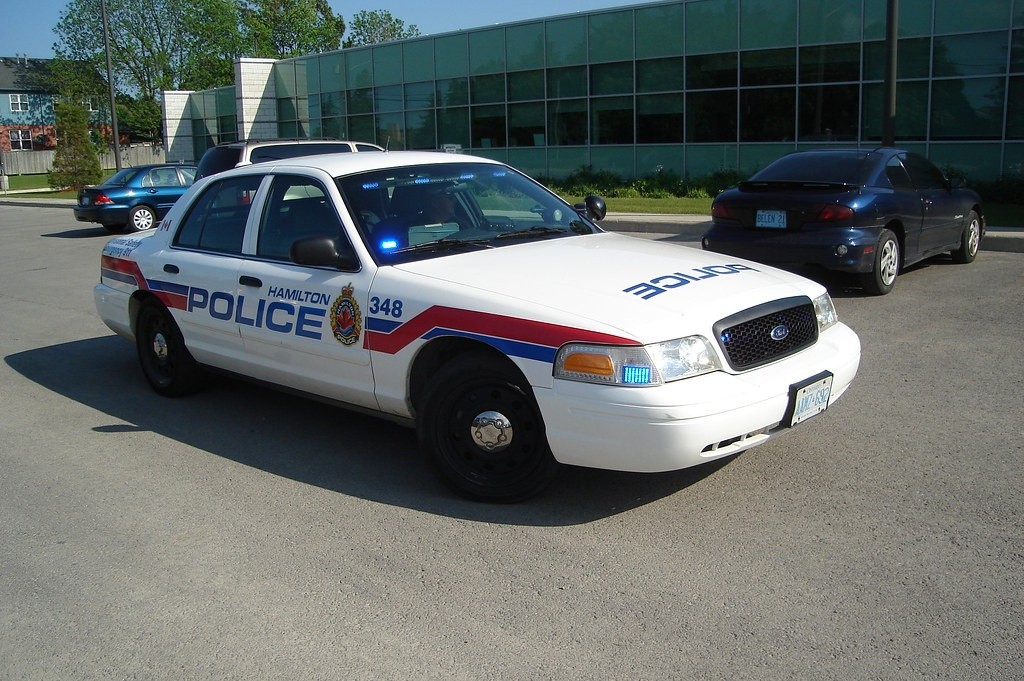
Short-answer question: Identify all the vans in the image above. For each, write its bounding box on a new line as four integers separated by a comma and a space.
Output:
190, 137, 401, 218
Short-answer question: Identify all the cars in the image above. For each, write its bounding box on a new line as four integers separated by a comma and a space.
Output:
701, 148, 986, 293
75, 163, 202, 233
92, 147, 864, 501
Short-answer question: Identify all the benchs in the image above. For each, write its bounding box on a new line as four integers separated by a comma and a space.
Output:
192, 199, 292, 252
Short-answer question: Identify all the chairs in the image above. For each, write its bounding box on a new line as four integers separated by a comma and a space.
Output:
152, 173, 164, 186
399, 194, 473, 246
165, 173, 179, 186
270, 198, 339, 267
